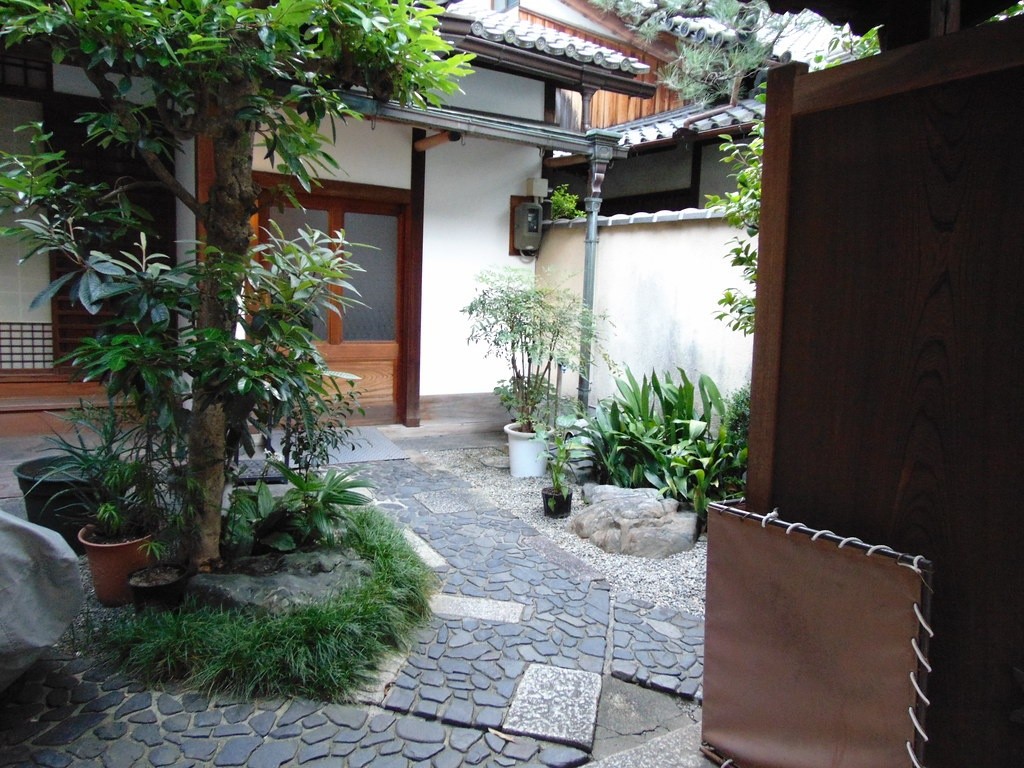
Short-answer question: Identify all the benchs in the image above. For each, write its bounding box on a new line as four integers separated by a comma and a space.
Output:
0, 394, 145, 436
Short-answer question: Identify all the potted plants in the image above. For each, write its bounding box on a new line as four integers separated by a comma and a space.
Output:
462, 264, 624, 478
14, 455, 111, 555
529, 424, 573, 519
40, 398, 153, 607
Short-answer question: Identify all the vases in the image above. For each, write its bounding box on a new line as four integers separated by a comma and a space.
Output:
127, 562, 188, 610
